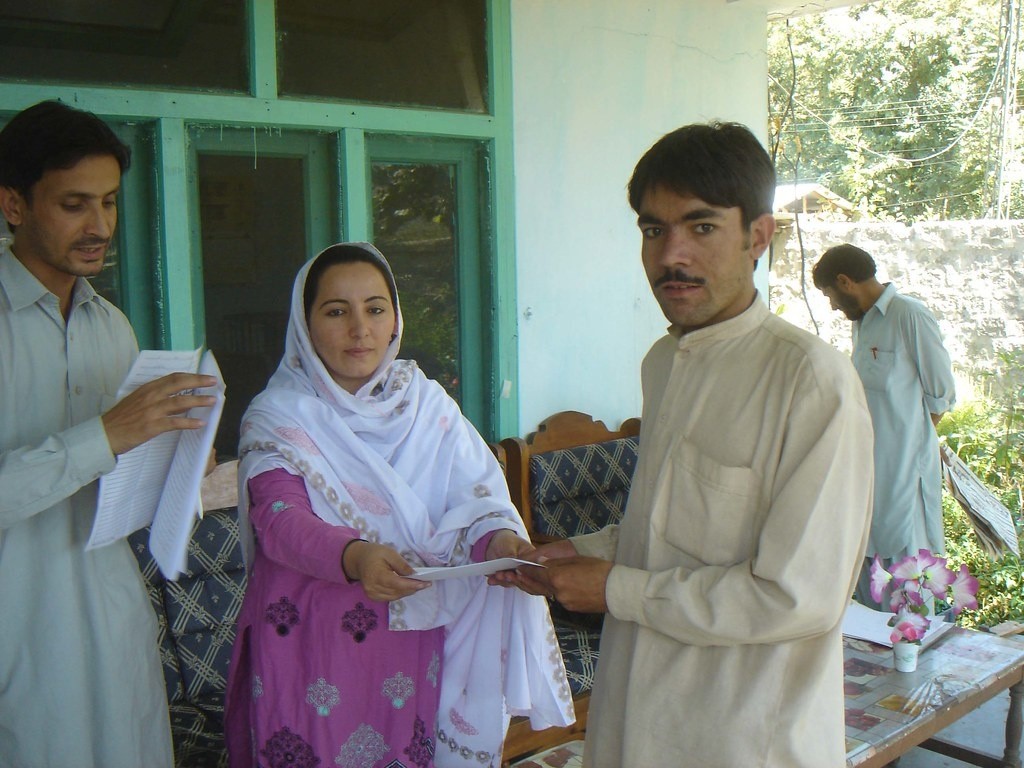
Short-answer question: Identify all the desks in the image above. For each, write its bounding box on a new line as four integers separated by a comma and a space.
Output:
507, 603, 1024, 768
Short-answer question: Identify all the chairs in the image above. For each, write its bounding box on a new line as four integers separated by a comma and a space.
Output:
129, 408, 641, 768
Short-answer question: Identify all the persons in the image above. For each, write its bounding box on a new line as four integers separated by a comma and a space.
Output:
222, 242, 535, 768
0, 98, 217, 768
508, 123, 875, 768
812, 242, 957, 625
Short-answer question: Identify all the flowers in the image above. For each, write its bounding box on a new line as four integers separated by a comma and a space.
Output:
869, 549, 982, 643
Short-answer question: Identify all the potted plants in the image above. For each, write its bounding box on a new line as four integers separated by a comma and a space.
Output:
890, 643, 922, 674
